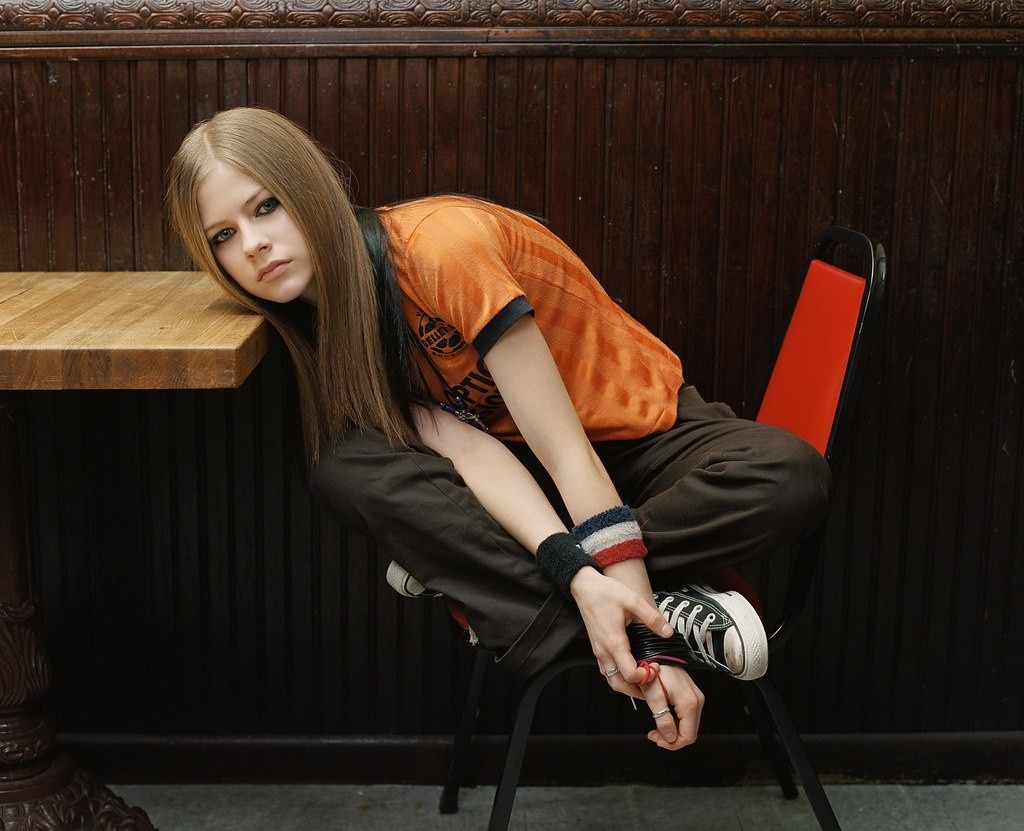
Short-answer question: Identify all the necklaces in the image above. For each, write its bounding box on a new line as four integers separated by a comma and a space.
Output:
407, 332, 489, 434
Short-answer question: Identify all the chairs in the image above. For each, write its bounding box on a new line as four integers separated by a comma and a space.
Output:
432, 222, 886, 831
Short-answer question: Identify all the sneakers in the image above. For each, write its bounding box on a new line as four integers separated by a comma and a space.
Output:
653, 583, 768, 680
387, 561, 444, 599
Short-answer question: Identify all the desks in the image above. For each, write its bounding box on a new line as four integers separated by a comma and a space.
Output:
0, 270, 270, 831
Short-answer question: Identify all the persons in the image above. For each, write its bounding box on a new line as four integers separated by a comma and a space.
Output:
168, 108, 831, 753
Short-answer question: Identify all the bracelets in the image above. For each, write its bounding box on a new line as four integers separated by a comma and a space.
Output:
535, 505, 649, 586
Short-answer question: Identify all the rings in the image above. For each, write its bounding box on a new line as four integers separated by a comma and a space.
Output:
604, 668, 619, 678
652, 708, 669, 719
